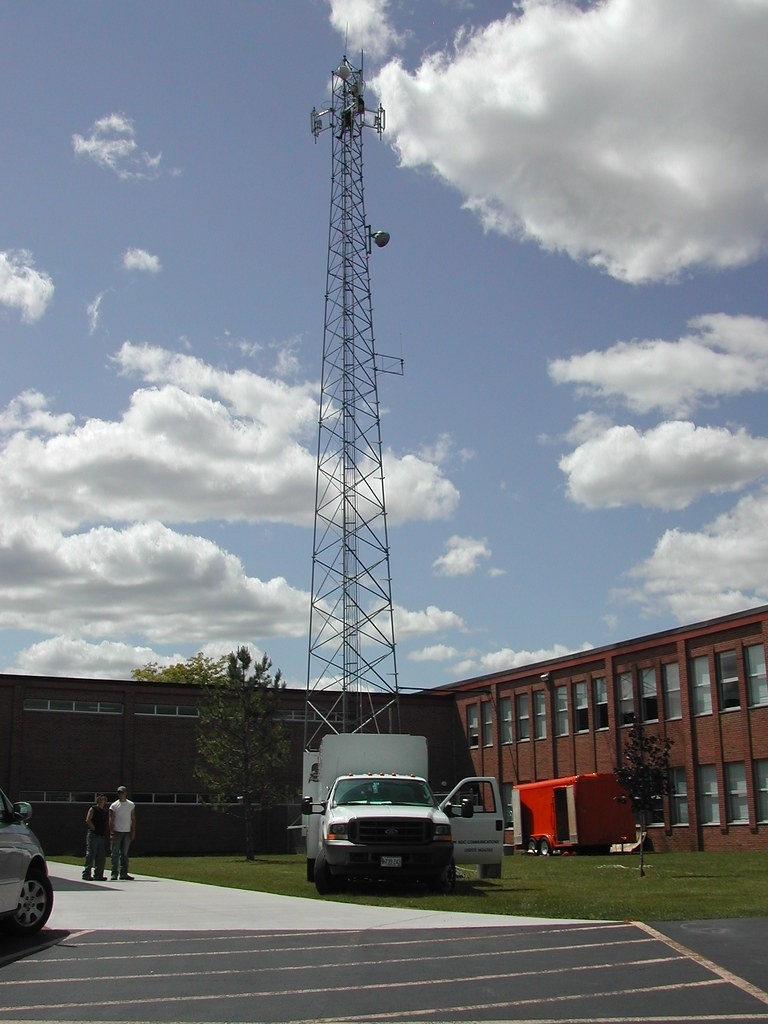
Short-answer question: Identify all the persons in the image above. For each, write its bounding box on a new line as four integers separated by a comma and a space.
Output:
82, 794, 109, 880
15, 805, 28, 819
108, 786, 135, 880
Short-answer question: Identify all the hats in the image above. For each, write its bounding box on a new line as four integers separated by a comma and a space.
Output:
97, 793, 105, 797
117, 786, 128, 792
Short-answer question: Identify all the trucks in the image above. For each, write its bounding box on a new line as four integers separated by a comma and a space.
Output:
301, 732, 505, 894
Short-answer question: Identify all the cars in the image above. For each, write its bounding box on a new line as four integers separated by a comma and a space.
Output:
0, 789, 53, 933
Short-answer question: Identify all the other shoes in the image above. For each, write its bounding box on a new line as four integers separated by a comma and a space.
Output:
82, 874, 94, 881
93, 875, 108, 881
111, 875, 117, 880
119, 873, 134, 881
